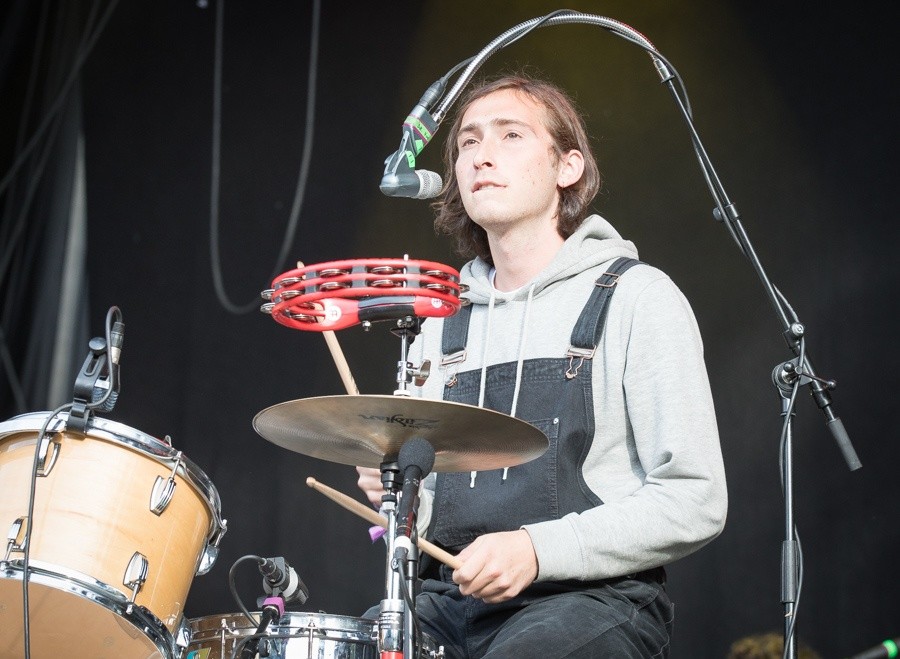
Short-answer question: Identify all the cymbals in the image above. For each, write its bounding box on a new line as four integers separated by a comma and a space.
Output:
259, 257, 472, 332
251, 394, 552, 475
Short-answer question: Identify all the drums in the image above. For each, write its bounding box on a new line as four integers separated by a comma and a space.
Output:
179, 609, 445, 659
0, 410, 229, 659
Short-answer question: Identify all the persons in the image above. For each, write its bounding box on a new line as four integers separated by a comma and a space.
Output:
357, 77, 728, 659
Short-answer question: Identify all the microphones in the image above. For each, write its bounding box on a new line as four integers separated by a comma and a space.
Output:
393, 436, 436, 563
857, 639, 900, 659
257, 557, 309, 607
380, 169, 443, 199
91, 320, 125, 412
